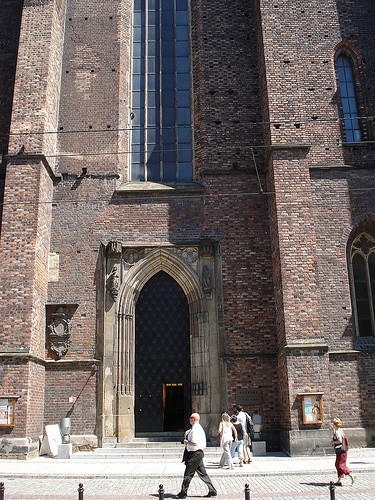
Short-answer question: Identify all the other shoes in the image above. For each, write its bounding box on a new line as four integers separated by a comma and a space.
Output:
334, 482, 342, 486
173, 495, 186, 499
204, 493, 217, 497
239, 460, 246, 464
239, 464, 243, 467
248, 459, 252, 463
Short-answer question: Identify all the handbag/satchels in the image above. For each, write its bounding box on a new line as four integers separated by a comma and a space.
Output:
217, 424, 223, 443
333, 436, 346, 454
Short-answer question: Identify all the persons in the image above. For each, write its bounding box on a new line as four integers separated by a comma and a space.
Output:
236, 405, 252, 464
203, 267, 211, 287
218, 413, 238, 469
172, 413, 217, 498
112, 267, 119, 289
331, 418, 355, 485
230, 415, 244, 467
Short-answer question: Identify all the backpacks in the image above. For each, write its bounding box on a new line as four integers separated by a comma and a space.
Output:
245, 412, 254, 436
